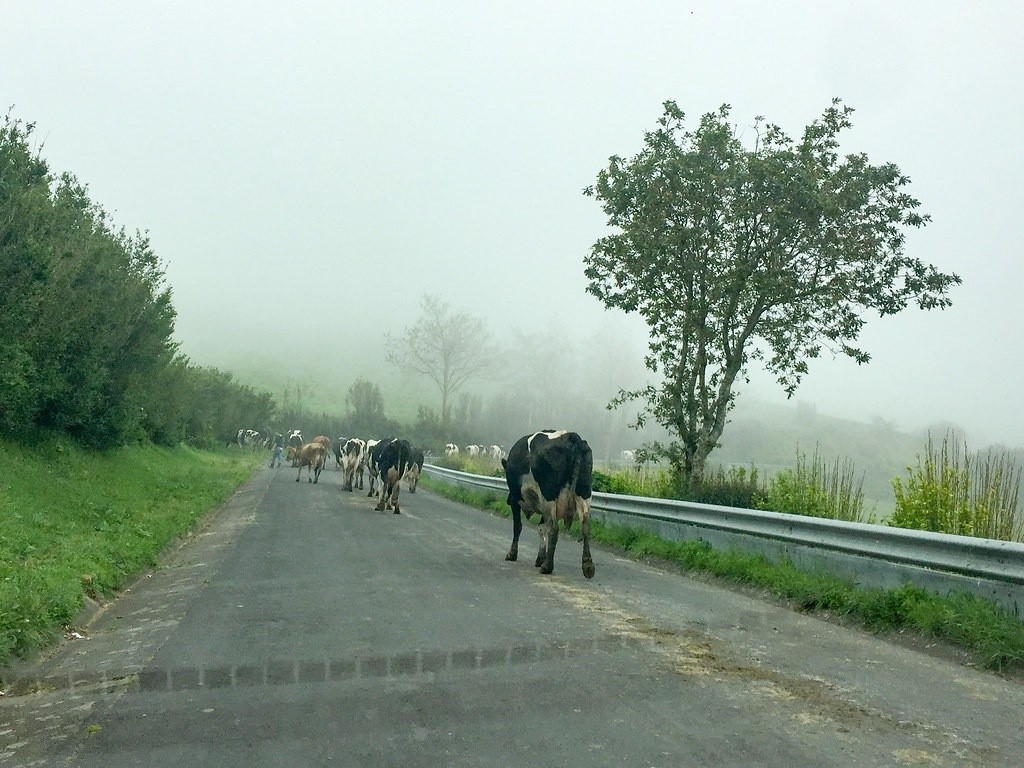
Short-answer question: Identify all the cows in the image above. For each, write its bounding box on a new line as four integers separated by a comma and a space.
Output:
621, 449, 635, 460
226, 428, 596, 579
